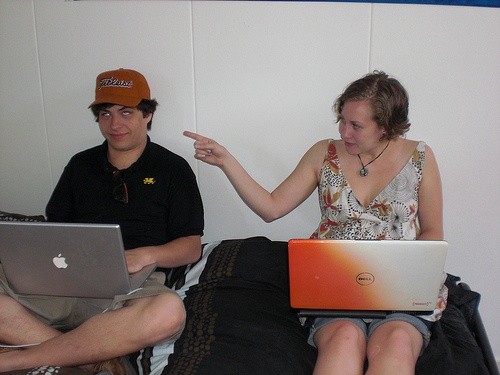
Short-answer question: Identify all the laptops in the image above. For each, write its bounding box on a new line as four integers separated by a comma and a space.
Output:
287, 239, 449, 319
0, 221, 159, 299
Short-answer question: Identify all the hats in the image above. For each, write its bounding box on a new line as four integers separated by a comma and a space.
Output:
88, 68, 150, 108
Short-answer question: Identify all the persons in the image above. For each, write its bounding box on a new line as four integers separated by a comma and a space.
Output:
0, 68, 204, 375
183, 71, 449, 375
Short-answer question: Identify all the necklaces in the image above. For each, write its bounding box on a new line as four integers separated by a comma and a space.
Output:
357, 139, 390, 177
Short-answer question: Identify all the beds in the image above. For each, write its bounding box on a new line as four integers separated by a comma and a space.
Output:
1, 211, 500, 375
